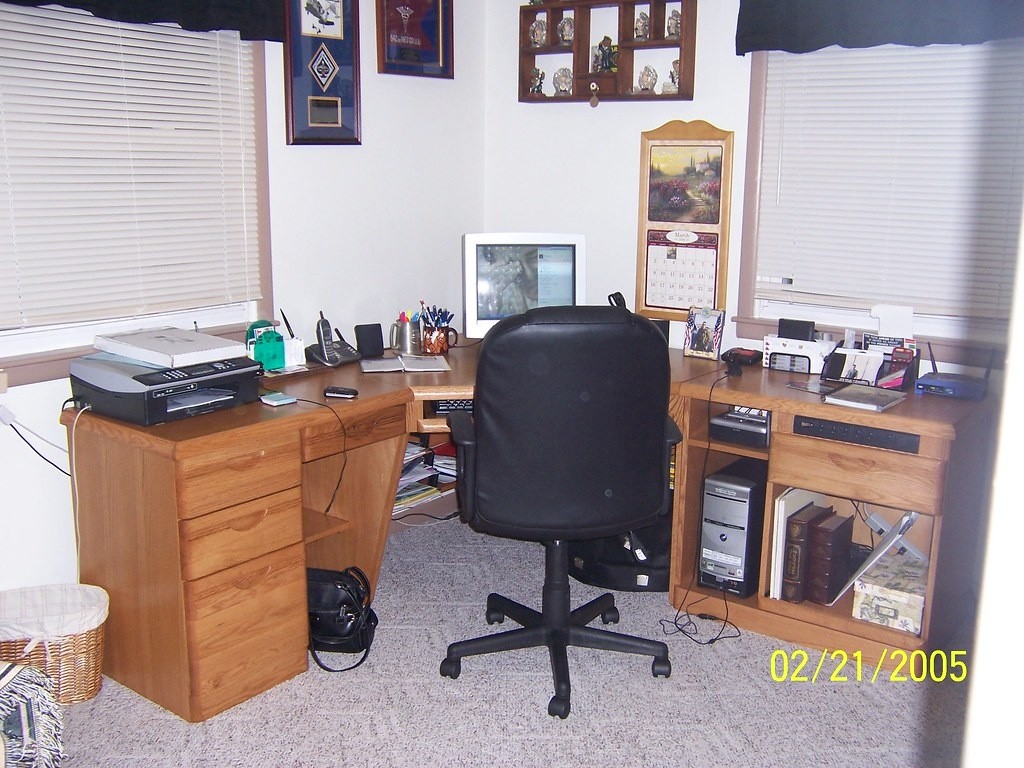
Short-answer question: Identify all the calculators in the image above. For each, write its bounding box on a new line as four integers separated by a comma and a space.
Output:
889, 347, 913, 374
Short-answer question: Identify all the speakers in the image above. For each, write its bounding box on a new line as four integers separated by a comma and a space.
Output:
355, 324, 384, 357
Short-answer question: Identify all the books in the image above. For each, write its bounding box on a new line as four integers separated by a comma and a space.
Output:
392, 442, 457, 516
359, 354, 452, 372
822, 383, 908, 413
769, 487, 855, 605
262, 392, 297, 406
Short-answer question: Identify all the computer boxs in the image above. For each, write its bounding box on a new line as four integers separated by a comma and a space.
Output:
696, 455, 769, 597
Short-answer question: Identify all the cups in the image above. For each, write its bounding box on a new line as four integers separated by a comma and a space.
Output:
423, 325, 458, 355
389, 319, 422, 355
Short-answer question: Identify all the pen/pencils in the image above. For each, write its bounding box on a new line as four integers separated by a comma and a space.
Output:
420, 300, 454, 327
280, 309, 295, 338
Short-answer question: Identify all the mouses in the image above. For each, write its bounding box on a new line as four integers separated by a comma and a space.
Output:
725, 359, 742, 376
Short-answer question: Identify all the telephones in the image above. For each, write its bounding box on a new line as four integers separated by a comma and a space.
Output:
304, 311, 362, 368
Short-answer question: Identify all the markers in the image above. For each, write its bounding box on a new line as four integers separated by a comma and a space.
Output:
399, 310, 420, 322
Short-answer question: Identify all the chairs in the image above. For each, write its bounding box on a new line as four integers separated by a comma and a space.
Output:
439, 305, 683, 720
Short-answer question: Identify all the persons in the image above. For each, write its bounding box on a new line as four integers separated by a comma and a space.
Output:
845, 364, 858, 379
694, 321, 709, 351
482, 245, 538, 318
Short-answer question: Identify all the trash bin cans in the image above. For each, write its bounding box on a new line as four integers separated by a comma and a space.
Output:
0, 585, 109, 706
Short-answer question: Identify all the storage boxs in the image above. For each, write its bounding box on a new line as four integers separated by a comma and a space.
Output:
762, 333, 835, 374
852, 552, 930, 636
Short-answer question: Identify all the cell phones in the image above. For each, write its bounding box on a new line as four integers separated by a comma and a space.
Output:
324, 386, 358, 398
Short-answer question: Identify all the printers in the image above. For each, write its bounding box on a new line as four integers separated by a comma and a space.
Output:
69, 350, 264, 427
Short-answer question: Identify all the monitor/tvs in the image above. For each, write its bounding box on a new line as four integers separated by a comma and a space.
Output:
462, 233, 587, 339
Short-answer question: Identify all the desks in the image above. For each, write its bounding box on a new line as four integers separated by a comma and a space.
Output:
332, 330, 728, 537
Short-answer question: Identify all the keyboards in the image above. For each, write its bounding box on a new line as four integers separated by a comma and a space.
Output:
432, 399, 474, 416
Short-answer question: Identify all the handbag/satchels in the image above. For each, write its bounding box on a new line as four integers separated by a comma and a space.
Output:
305, 565, 379, 673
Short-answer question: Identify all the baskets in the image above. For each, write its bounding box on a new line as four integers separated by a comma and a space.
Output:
0, 583, 110, 705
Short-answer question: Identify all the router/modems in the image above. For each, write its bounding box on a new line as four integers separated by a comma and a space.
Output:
914, 341, 996, 400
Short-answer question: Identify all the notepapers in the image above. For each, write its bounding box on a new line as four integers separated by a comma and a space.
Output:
259, 392, 297, 406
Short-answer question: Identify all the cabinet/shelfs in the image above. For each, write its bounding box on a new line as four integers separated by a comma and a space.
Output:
667, 359, 996, 679
59, 371, 416, 723
518, 0, 699, 108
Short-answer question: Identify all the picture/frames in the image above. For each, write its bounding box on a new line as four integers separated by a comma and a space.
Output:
375, 0, 455, 80
683, 306, 726, 360
283, 0, 363, 145
